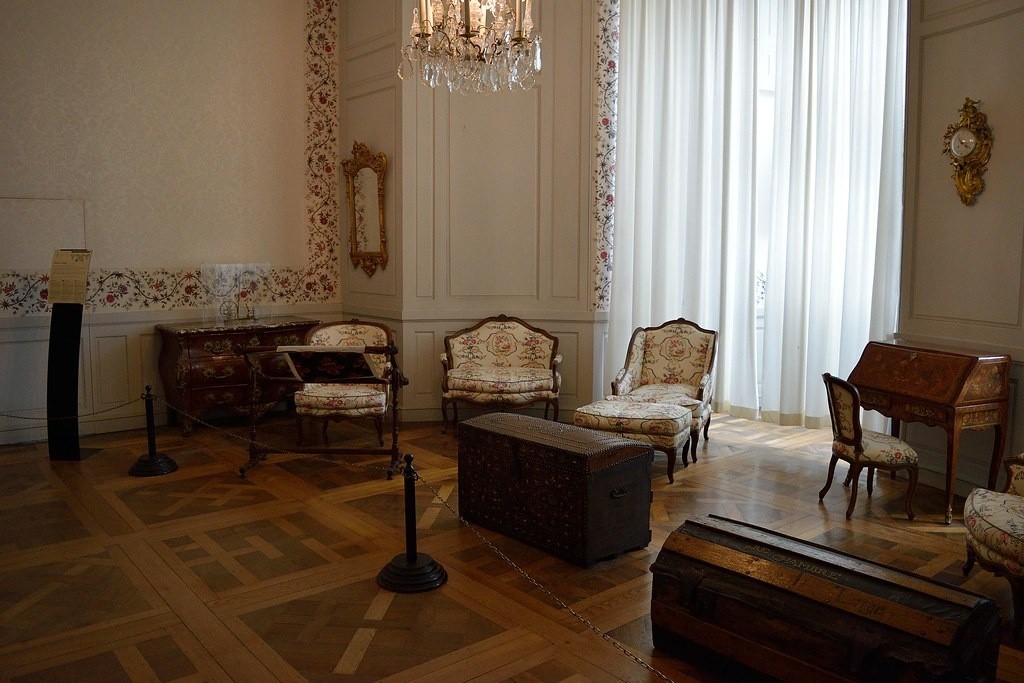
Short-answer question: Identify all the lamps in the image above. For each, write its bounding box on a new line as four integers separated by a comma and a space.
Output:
395, 0, 545, 94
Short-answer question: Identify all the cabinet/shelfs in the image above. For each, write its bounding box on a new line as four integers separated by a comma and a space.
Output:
155, 316, 320, 437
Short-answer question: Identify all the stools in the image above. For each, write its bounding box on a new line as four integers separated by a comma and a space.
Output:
574, 398, 694, 483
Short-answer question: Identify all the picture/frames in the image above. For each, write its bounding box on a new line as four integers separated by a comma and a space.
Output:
340, 139, 390, 277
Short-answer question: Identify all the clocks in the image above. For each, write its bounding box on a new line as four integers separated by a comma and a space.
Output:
940, 97, 993, 205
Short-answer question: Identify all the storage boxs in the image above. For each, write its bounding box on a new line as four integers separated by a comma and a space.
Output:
455, 409, 655, 570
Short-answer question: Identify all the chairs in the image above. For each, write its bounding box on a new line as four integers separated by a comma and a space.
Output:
963, 452, 1024, 641
294, 317, 394, 445
820, 372, 919, 522
610, 317, 719, 460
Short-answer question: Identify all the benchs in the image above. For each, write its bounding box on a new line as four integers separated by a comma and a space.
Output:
435, 314, 562, 433
